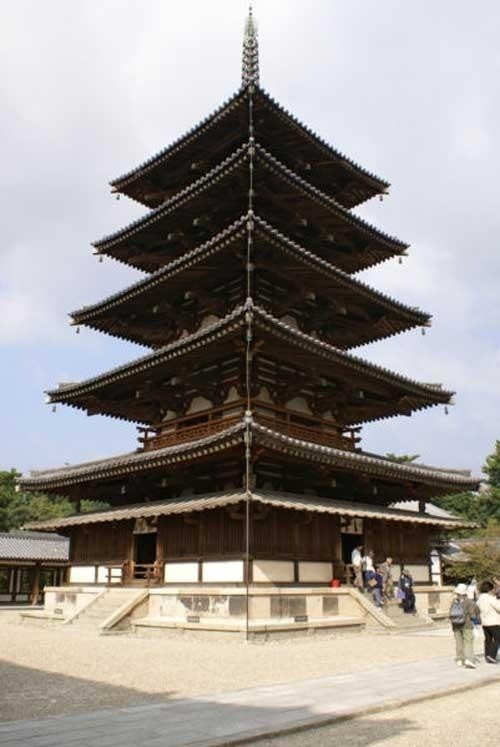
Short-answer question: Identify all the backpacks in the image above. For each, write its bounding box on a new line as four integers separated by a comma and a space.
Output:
449, 599, 466, 625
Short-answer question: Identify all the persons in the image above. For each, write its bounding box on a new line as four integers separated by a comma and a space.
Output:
448, 573, 500, 670
351, 541, 417, 614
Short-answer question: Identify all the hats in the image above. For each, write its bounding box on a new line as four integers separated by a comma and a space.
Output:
402, 568, 411, 575
454, 583, 468, 596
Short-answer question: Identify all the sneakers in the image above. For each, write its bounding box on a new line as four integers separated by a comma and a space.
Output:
457, 660, 475, 668
486, 655, 497, 663
